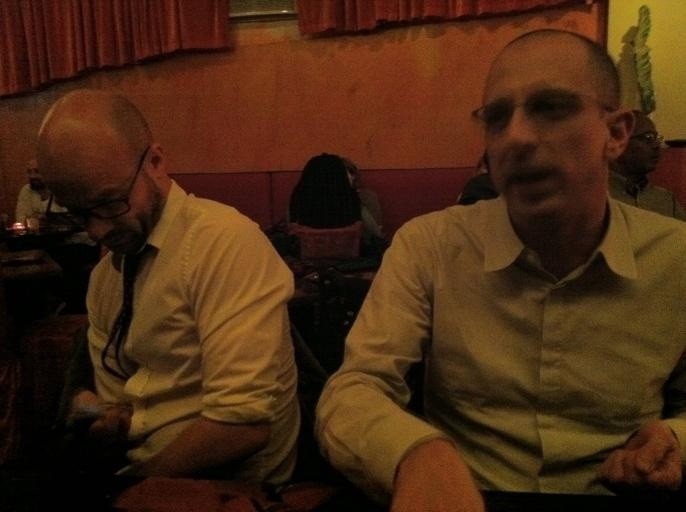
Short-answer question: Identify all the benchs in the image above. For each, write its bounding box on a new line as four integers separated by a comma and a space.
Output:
270, 166, 487, 248
646, 147, 686, 222
166, 172, 272, 235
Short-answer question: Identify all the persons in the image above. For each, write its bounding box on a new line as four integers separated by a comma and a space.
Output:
34, 89, 301, 511
313, 30, 686, 510
13, 157, 101, 264
604, 109, 684, 219
339, 152, 387, 254
451, 146, 499, 204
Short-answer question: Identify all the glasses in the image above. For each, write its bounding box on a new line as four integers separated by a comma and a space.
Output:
45, 148, 148, 227
470, 90, 610, 124
632, 132, 665, 145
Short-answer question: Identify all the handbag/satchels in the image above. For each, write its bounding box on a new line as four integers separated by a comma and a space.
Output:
288, 152, 358, 227
